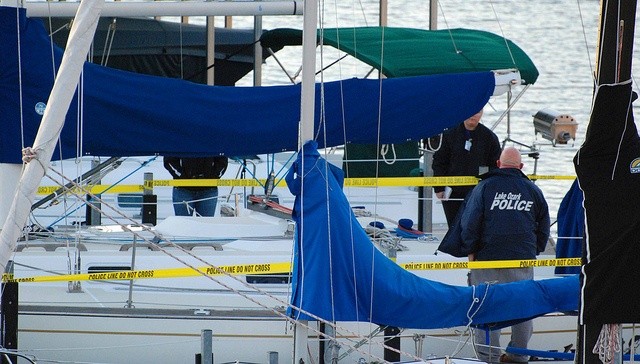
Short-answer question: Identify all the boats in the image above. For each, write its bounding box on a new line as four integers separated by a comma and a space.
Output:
0, 160, 640, 364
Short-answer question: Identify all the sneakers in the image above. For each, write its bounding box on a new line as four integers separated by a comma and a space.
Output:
500, 354, 528, 364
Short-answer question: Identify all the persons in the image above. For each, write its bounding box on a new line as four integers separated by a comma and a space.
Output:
162, 156, 228, 217
461, 147, 550, 363
433, 108, 502, 228
